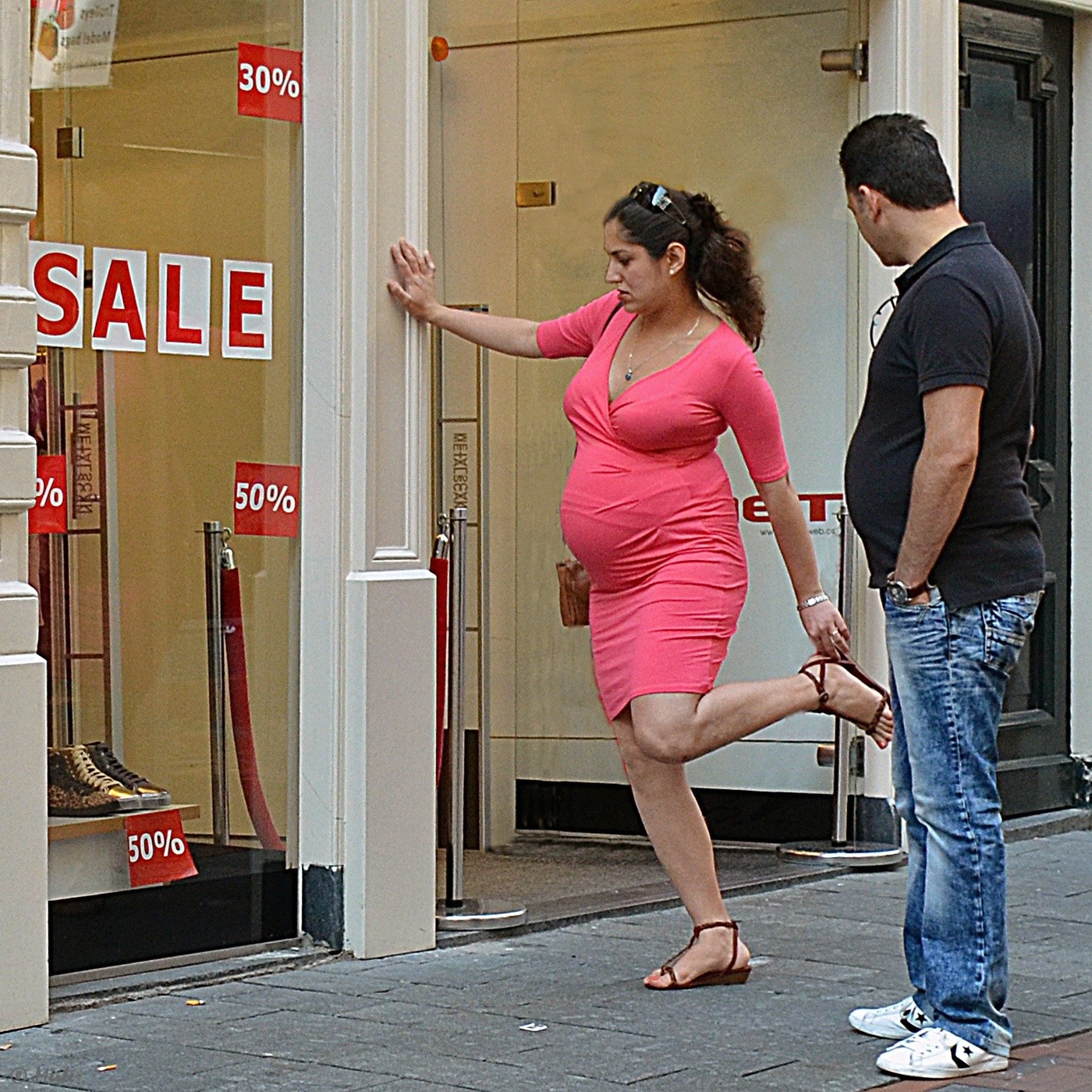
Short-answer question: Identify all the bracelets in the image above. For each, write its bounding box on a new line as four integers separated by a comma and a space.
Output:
796, 595, 829, 610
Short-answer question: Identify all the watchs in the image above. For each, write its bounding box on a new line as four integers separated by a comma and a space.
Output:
886, 571, 928, 604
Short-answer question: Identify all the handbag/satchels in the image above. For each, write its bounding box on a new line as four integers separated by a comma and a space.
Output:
556, 560, 590, 627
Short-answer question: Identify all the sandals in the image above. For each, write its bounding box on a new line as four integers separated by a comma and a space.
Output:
644, 920, 751, 990
799, 643, 894, 749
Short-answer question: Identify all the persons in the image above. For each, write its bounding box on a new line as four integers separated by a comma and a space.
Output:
385, 180, 892, 990
839, 112, 1046, 1080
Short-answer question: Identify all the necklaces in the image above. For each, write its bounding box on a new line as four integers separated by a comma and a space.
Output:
625, 314, 699, 380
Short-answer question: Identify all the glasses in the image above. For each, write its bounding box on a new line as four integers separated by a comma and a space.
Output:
630, 181, 693, 247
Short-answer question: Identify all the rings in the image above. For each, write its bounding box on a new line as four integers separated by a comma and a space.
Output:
830, 630, 838, 635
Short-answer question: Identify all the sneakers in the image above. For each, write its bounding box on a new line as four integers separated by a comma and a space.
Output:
60, 745, 142, 812
84, 742, 171, 809
848, 996, 935, 1039
876, 1027, 1008, 1079
48, 748, 119, 818
